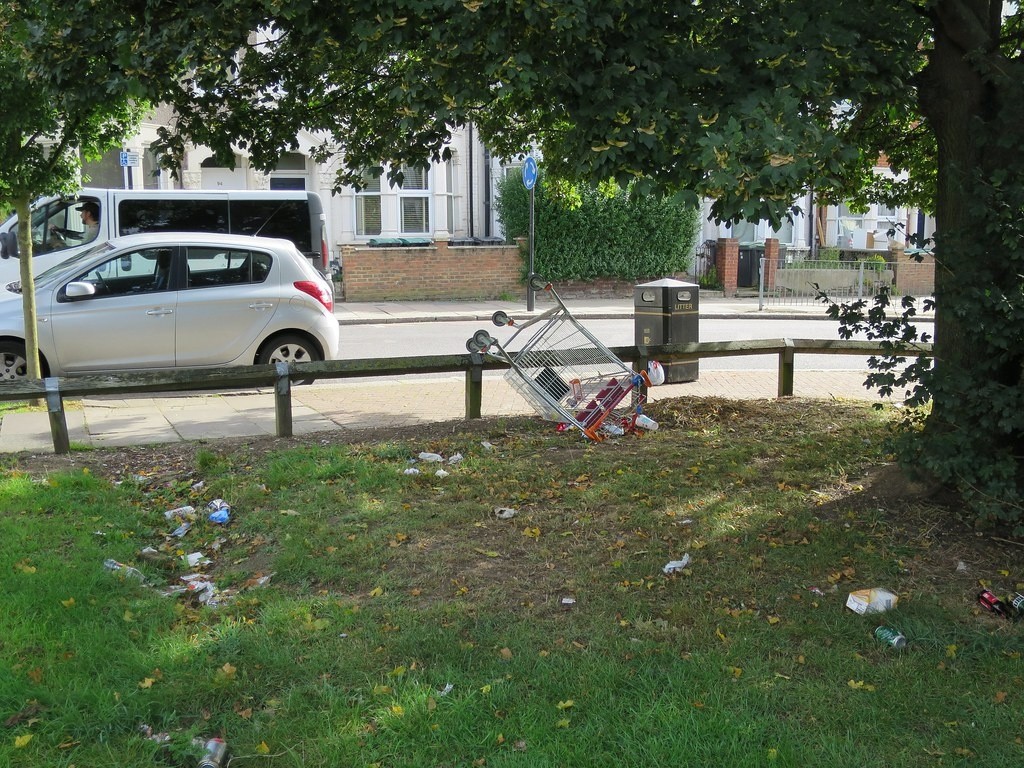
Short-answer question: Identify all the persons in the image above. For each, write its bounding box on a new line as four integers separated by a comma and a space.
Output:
128, 251, 172, 293
48, 202, 99, 249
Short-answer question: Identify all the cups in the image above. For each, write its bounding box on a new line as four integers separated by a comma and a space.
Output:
634, 414, 659, 430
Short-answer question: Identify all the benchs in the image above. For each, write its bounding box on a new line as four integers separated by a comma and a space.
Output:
233, 262, 267, 283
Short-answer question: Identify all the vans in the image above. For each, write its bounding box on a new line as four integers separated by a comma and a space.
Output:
0, 187, 335, 297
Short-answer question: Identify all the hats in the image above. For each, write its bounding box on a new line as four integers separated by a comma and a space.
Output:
75, 203, 99, 214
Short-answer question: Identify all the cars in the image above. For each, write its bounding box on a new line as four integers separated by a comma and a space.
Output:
0, 231, 338, 388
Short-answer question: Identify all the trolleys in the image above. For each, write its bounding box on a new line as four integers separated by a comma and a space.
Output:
465, 270, 652, 447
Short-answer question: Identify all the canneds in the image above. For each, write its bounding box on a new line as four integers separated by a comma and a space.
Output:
196, 737, 228, 768
1005, 590, 1024, 615
875, 625, 906, 648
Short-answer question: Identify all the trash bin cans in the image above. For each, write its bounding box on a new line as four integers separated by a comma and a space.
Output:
633, 277, 699, 383
366, 235, 505, 247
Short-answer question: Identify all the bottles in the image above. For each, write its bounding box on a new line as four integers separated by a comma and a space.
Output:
1006, 592, 1024, 616
103, 558, 146, 585
976, 589, 1015, 621
